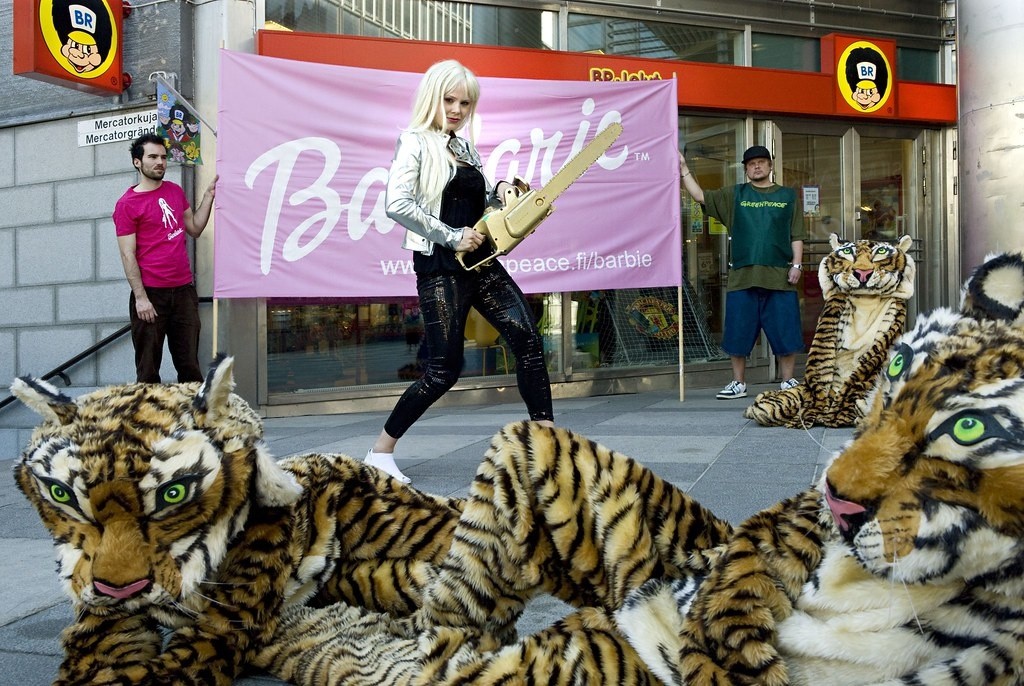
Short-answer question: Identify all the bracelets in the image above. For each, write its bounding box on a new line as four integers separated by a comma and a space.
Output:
793, 264, 804, 271
681, 171, 691, 177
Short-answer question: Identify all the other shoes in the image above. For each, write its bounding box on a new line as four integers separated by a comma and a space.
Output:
361, 448, 411, 484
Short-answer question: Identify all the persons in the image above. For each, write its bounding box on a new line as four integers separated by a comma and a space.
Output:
678, 146, 811, 400
111, 135, 220, 385
363, 58, 554, 484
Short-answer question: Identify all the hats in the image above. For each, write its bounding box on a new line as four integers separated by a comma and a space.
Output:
742, 146, 771, 162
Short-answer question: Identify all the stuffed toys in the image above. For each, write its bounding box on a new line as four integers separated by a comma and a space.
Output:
10, 352, 532, 685
744, 228, 920, 431
393, 253, 1024, 685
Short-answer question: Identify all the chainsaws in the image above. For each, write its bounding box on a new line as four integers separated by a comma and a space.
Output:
453, 122, 625, 273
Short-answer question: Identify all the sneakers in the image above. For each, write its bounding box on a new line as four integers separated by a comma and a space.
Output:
716, 380, 748, 399
779, 377, 799, 390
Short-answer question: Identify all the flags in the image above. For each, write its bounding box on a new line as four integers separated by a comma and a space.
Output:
154, 81, 204, 166
